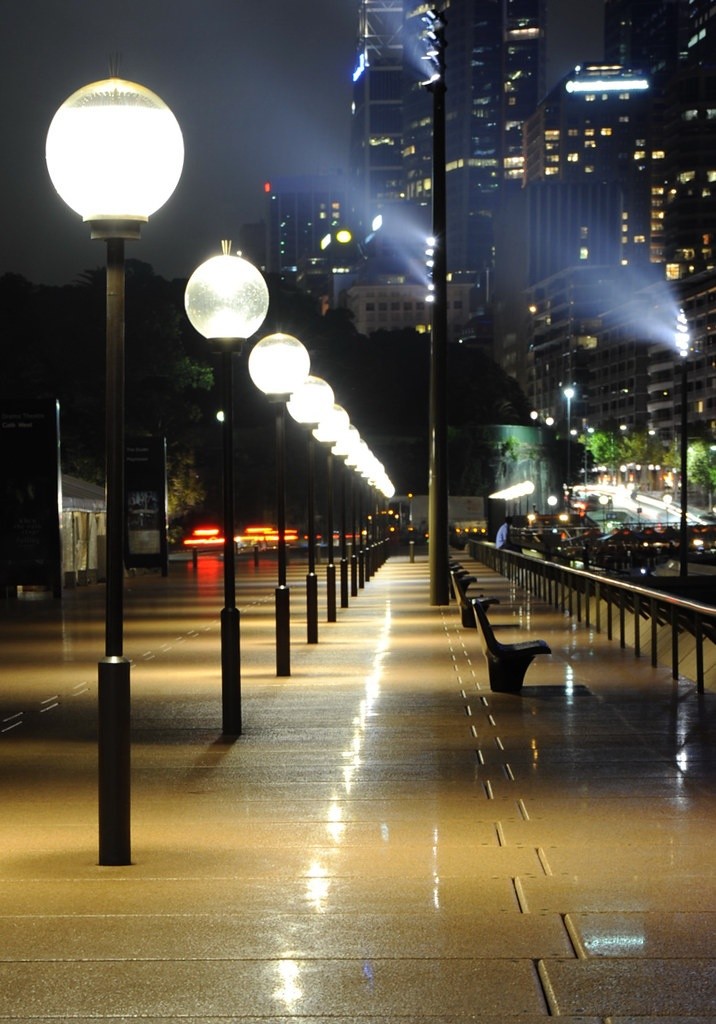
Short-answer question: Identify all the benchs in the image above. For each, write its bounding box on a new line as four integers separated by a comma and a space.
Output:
448, 551, 552, 693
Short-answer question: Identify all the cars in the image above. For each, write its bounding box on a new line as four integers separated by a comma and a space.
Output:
673, 524, 716, 562
510, 513, 600, 560
585, 528, 677, 573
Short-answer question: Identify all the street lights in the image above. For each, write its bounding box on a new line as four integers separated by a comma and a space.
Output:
563, 388, 574, 521
599, 496, 609, 534
679, 349, 693, 583
248, 332, 311, 678
547, 496, 557, 521
183, 239, 271, 733
663, 494, 672, 528
286, 374, 395, 643
488, 482, 535, 516
45, 78, 184, 869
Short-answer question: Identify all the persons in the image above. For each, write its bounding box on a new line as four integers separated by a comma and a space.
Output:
495, 516, 521, 553
